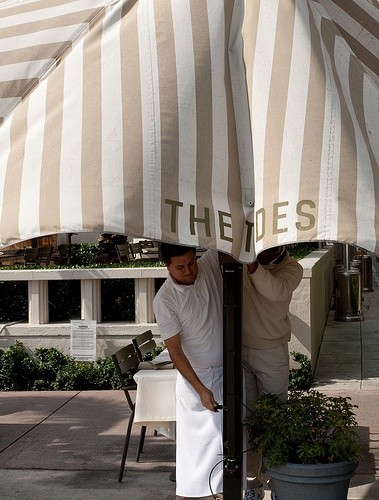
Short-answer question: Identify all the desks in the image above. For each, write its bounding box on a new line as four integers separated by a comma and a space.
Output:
141, 246, 207, 263
133, 348, 177, 483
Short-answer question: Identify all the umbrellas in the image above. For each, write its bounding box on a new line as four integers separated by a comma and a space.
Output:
0, 0, 379, 500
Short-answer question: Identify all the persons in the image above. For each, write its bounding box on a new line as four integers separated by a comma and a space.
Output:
151, 243, 226, 500
244, 245, 303, 500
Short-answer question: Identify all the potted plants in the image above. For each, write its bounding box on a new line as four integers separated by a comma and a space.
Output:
242, 389, 364, 500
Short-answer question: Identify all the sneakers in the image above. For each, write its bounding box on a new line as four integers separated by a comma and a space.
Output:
270, 490, 280, 500
244, 484, 266, 500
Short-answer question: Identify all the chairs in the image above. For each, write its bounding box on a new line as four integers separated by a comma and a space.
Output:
111, 343, 147, 483
0, 239, 154, 267
132, 329, 158, 363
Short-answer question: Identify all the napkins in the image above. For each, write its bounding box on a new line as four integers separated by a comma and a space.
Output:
137, 361, 176, 370
